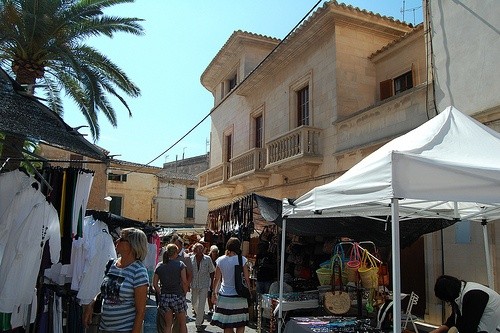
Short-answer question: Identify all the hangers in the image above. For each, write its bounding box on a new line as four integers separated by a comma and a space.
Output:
18, 161, 108, 222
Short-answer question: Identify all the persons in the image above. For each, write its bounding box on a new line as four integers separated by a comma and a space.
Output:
211, 237, 252, 333
83, 227, 150, 333
187, 242, 216, 332
146, 231, 210, 302
207, 245, 219, 315
254, 241, 278, 319
153, 244, 190, 333
262, 273, 293, 326
429, 275, 500, 333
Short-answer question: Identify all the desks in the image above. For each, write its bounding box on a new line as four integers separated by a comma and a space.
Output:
282, 317, 357, 333
261, 294, 410, 333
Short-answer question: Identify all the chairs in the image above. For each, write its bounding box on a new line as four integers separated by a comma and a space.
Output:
401, 291, 419, 333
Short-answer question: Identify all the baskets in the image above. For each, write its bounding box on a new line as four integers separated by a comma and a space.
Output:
316, 243, 390, 288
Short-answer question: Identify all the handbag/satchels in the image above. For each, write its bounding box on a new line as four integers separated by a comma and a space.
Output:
93, 293, 107, 314
323, 291, 351, 315
235, 255, 251, 298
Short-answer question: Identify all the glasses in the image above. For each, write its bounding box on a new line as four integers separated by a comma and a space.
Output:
119, 236, 128, 241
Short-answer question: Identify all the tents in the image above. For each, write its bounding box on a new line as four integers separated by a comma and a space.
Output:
277, 105, 500, 333
